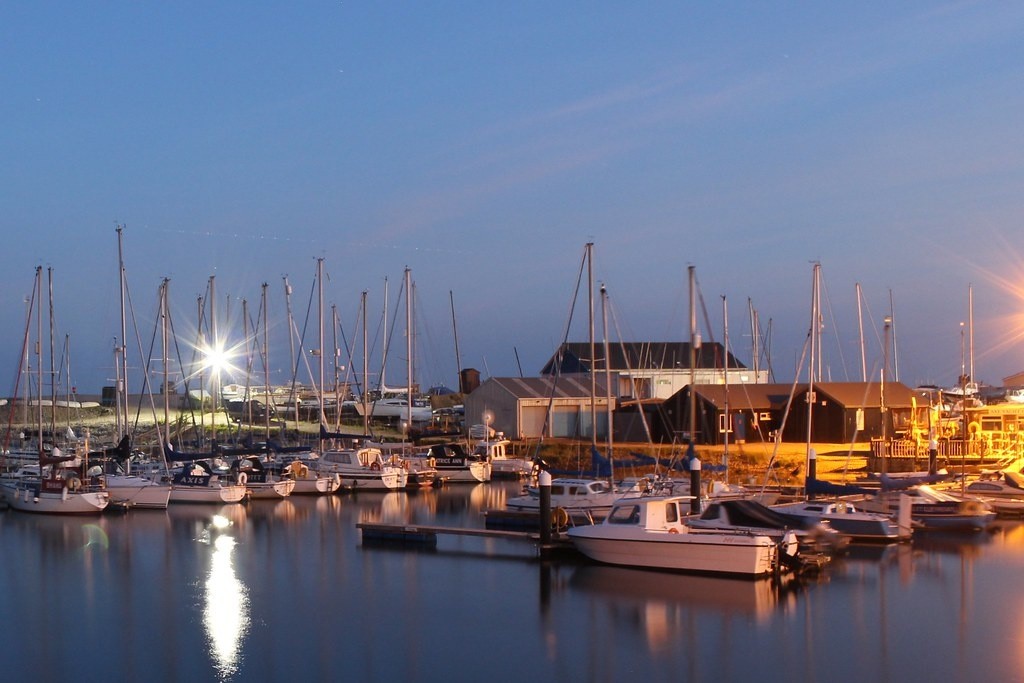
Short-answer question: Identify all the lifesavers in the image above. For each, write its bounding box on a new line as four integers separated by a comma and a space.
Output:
707, 478, 715, 494
238, 472, 247, 484
68, 477, 82, 490
670, 527, 681, 535
298, 467, 307, 478
371, 462, 380, 471
551, 507, 568, 526
429, 457, 436, 467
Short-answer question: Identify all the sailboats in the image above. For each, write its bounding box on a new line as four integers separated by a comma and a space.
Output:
0, 239, 1024, 574
